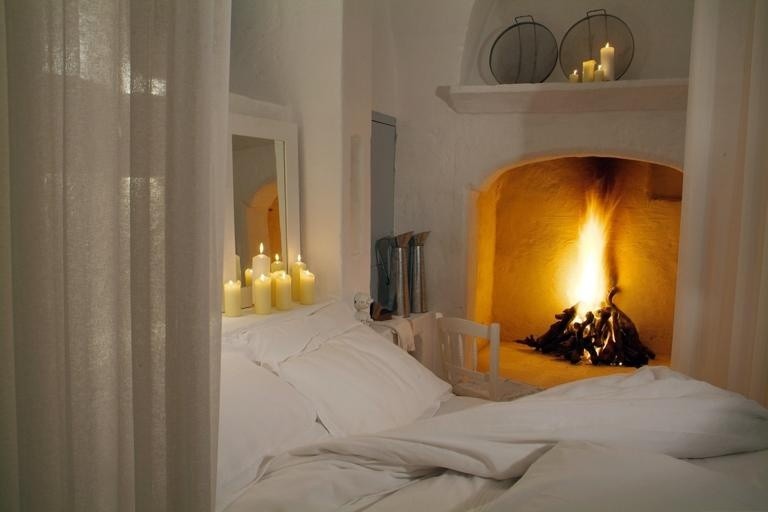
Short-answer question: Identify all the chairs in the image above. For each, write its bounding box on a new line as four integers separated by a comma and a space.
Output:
437, 312, 540, 401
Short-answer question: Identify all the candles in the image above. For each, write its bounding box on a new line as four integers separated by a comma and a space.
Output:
570, 42, 615, 82
224, 242, 316, 317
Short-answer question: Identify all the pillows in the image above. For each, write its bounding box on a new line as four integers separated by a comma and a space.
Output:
214, 350, 331, 509
224, 302, 363, 372
268, 323, 455, 440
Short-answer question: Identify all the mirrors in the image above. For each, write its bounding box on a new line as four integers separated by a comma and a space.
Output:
370, 120, 396, 311
224, 114, 300, 309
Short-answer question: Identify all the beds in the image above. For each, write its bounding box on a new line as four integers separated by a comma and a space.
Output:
214, 301, 768, 512
